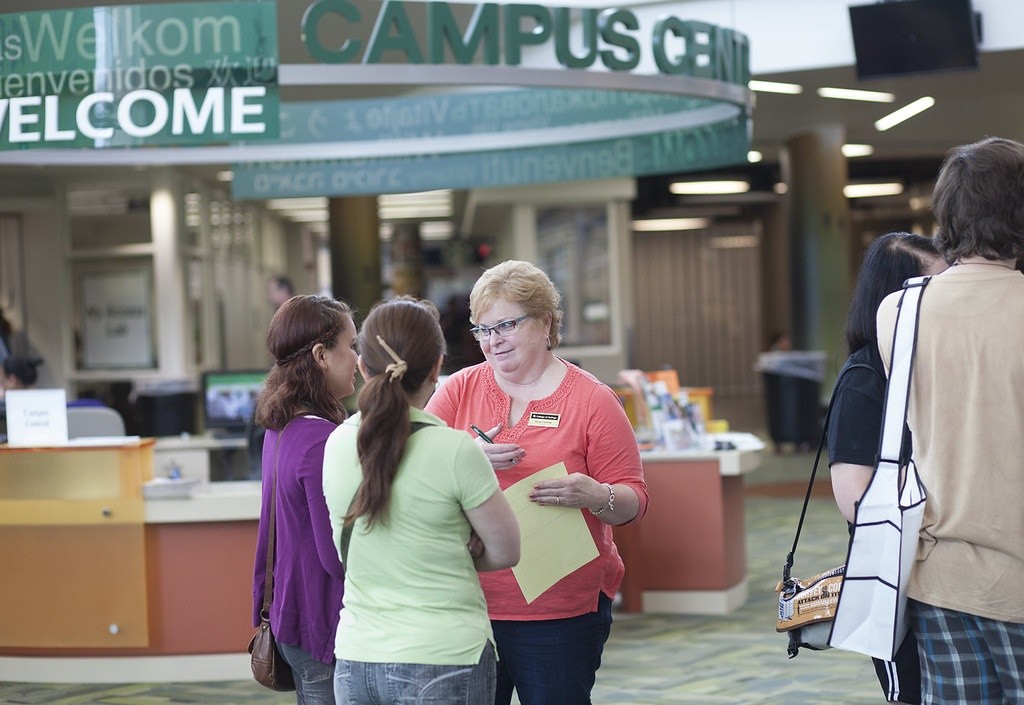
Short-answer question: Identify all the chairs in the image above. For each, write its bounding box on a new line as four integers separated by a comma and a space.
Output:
65, 407, 127, 437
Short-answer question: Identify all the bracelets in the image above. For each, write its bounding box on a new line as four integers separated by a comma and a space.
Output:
588, 483, 614, 515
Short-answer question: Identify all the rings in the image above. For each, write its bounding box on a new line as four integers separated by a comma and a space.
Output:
556, 497, 559, 504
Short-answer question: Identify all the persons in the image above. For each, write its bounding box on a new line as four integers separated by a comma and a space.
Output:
829, 137, 1024, 705
322, 295, 520, 705
253, 295, 358, 705
423, 260, 649, 705
0, 304, 39, 434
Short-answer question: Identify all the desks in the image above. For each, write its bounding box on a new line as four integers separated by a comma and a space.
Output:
151, 430, 250, 483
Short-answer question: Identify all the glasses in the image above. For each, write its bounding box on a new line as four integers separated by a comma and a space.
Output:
468, 312, 531, 341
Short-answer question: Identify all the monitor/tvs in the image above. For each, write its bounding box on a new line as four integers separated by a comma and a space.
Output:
201, 369, 269, 440
848, 0, 980, 78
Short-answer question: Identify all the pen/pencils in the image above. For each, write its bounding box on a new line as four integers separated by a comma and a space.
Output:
468, 424, 518, 466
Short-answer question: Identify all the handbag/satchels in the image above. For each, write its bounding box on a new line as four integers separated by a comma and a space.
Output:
246, 624, 297, 693
826, 463, 927, 663
775, 564, 845, 658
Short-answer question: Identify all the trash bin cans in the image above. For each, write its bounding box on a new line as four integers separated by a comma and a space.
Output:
754, 352, 825, 455
